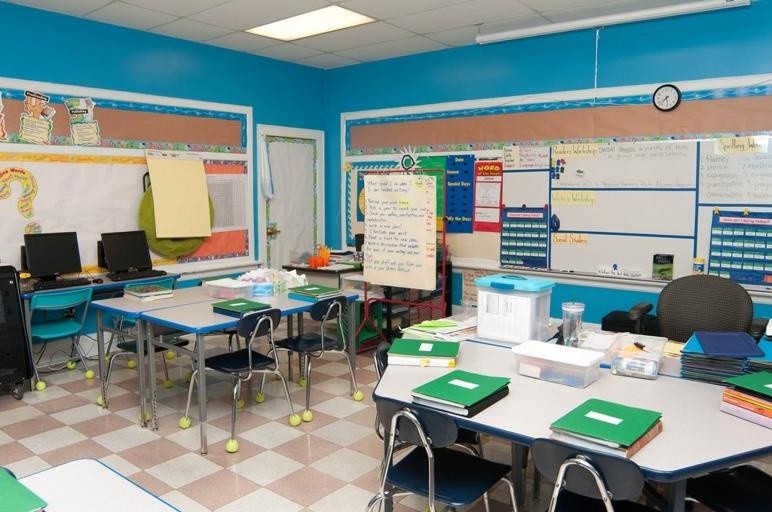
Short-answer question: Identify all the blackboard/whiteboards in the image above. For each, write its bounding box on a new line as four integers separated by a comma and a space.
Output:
340, 74, 772, 299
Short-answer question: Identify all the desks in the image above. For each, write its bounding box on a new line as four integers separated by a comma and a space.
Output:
16, 457, 181, 512
373, 310, 771, 512
19, 272, 181, 390
90, 286, 359, 456
282, 263, 363, 380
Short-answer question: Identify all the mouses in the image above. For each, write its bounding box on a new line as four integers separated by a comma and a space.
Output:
93, 279, 103, 283
160, 271, 167, 275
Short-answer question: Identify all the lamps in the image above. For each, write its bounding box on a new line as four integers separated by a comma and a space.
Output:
475, 0, 751, 45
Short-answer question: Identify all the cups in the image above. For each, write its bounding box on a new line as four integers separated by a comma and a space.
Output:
560, 301, 585, 349
308, 256, 329, 268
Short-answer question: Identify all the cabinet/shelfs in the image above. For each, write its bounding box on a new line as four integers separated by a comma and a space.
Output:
382, 260, 452, 345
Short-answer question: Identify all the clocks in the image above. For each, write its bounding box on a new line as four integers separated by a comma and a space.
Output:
652, 84, 682, 112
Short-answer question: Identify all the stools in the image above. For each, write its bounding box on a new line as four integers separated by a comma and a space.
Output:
602, 311, 656, 336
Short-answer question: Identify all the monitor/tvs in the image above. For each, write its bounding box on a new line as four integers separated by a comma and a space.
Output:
24, 231, 82, 281
101, 230, 152, 272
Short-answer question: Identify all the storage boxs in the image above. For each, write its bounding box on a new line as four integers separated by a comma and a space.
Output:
205, 278, 256, 300
473, 273, 556, 344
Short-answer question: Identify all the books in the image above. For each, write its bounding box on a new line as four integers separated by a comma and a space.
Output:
411, 370, 511, 417
287, 283, 343, 304
402, 318, 478, 342
387, 338, 460, 367
680, 330, 772, 431
318, 250, 362, 273
123, 284, 174, 303
211, 297, 272, 319
549, 398, 662, 459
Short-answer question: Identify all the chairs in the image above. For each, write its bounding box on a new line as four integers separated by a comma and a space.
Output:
105, 278, 175, 368
365, 396, 518, 512
685, 465, 772, 511
373, 346, 483, 458
629, 274, 769, 344
530, 438, 660, 512
256, 295, 363, 421
179, 308, 302, 453
30, 285, 94, 390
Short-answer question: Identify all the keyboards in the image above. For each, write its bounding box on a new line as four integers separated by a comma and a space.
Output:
107, 270, 161, 281
34, 278, 91, 291
